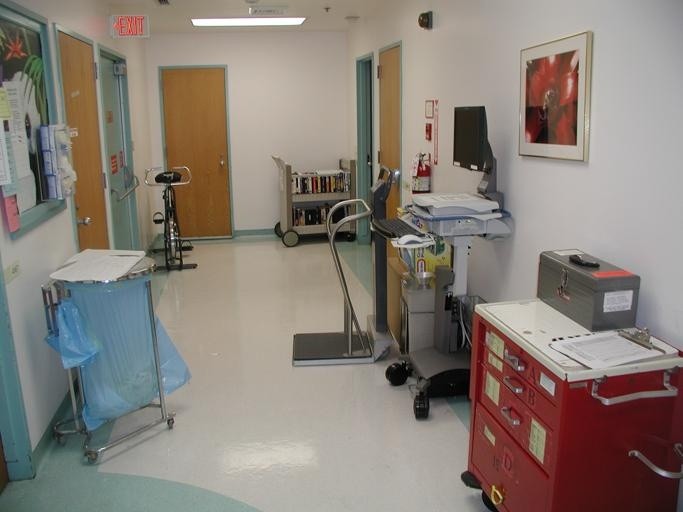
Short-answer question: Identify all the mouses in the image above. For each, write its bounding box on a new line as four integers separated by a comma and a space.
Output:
398, 233, 423, 244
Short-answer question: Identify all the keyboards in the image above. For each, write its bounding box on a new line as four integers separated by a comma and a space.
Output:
371, 216, 425, 237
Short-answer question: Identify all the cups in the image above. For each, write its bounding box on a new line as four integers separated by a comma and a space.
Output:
414, 271, 433, 286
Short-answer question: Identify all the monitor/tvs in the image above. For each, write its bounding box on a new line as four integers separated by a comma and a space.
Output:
452, 106, 494, 175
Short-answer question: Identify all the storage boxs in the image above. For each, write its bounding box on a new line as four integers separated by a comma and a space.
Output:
536, 248, 640, 332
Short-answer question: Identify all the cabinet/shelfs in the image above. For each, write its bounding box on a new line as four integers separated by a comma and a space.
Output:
460, 299, 682, 511
272, 156, 356, 247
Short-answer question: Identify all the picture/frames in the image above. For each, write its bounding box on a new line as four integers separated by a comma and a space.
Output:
1, 1, 68, 242
519, 31, 593, 162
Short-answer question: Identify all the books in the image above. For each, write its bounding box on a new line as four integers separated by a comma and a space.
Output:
292, 203, 350, 226
292, 168, 351, 194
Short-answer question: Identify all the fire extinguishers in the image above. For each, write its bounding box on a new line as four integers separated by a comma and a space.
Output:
413, 154, 432, 195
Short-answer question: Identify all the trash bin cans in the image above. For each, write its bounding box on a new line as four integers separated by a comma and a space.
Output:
55, 257, 190, 433
